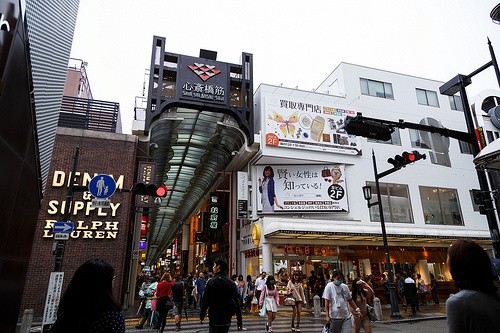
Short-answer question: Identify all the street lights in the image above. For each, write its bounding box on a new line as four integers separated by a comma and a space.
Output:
363, 148, 402, 319
439, 36, 500, 96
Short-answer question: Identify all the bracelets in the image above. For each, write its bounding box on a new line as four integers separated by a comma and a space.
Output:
354, 308, 360, 312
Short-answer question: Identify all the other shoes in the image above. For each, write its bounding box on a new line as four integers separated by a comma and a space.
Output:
153, 326, 158, 329
135, 325, 143, 329
176, 323, 180, 328
291, 326, 296, 331
268, 326, 272, 333
296, 325, 301, 332
265, 321, 269, 331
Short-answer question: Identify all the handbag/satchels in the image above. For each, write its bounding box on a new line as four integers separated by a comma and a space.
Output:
283, 290, 296, 306
322, 323, 331, 333
259, 301, 266, 317
251, 296, 258, 304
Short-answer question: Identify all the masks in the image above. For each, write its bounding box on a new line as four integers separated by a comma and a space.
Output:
333, 278, 343, 286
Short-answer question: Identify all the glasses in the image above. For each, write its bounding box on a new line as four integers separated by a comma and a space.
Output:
112, 274, 118, 281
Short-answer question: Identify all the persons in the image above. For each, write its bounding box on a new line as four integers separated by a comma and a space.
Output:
231, 274, 246, 310
446, 239, 500, 333
259, 275, 281, 332
49, 257, 126, 333
200, 258, 243, 333
152, 272, 174, 333
258, 166, 284, 212
430, 273, 440, 307
134, 271, 214, 330
303, 270, 429, 314
322, 271, 361, 333
287, 272, 307, 332
244, 275, 255, 311
349, 277, 374, 333
254, 272, 267, 309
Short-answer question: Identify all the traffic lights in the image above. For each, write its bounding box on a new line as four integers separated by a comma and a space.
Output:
128, 182, 168, 197
388, 150, 422, 167
